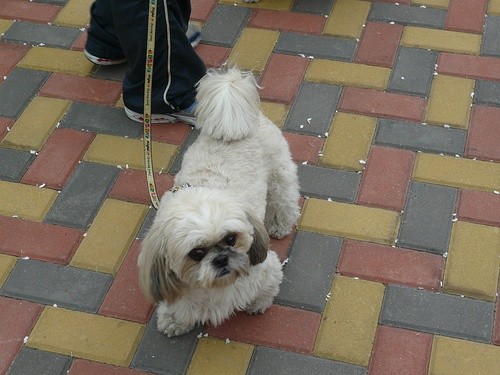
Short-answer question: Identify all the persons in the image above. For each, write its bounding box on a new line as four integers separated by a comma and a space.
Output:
83, 0, 206, 127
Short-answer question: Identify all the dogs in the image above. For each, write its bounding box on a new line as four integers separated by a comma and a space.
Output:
137, 63, 302, 338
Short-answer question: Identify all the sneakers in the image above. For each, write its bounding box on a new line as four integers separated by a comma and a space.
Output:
85, 23, 203, 64
123, 77, 201, 126
